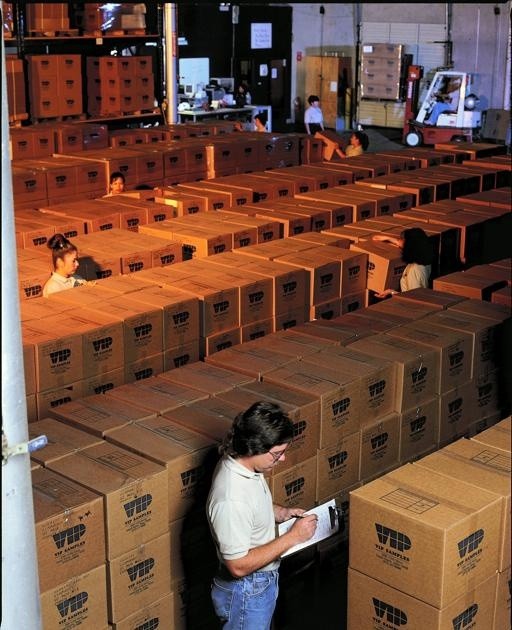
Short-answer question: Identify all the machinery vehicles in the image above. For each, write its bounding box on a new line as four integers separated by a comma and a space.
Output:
400, 58, 479, 148
175, 79, 277, 138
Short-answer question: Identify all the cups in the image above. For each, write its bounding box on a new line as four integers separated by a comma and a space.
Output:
204, 104, 209, 112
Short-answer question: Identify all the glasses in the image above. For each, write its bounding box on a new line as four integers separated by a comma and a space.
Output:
268, 448, 287, 459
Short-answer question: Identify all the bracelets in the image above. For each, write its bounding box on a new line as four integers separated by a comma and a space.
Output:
391, 290, 395, 295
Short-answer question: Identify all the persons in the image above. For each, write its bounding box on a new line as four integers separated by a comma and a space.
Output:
304, 95, 325, 136
424, 77, 461, 126
204, 400, 318, 630
234, 114, 269, 133
42, 233, 86, 298
103, 171, 125, 198
334, 131, 368, 158
235, 84, 252, 104
374, 227, 431, 299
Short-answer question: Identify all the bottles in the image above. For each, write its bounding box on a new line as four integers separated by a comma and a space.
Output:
246, 92, 251, 105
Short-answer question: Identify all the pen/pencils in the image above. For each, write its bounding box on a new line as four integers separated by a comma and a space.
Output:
291, 515, 318, 521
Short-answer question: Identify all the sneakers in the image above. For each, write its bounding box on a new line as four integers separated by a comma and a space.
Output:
424, 120, 435, 126
